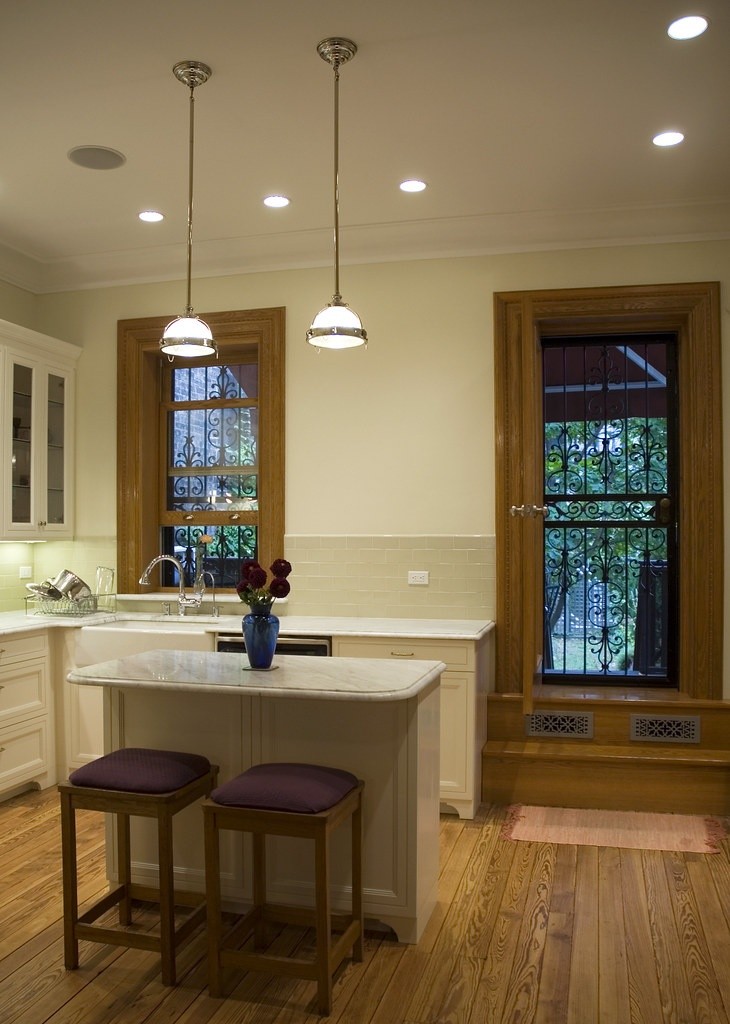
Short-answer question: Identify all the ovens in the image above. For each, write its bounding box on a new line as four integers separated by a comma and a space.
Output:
214, 632, 331, 657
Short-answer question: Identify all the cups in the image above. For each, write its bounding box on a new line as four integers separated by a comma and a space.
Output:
13, 417, 21, 438
95, 567, 114, 607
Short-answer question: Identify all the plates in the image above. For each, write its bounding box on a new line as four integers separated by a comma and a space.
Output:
25, 583, 62, 599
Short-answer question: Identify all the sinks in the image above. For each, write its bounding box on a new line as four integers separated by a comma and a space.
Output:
79, 611, 221, 668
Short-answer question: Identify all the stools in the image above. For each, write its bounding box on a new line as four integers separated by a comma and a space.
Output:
200, 764, 365, 1015
58, 748, 219, 988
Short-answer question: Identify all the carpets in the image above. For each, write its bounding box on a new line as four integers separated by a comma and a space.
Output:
504, 804, 725, 855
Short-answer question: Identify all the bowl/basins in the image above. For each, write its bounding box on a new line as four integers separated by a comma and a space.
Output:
54, 570, 91, 605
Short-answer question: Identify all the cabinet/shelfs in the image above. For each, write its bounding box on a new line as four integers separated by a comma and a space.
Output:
63, 664, 103, 785
0, 318, 84, 543
442, 671, 487, 819
67, 650, 447, 947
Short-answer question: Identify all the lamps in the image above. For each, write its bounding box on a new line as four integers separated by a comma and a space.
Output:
159, 59, 218, 361
306, 39, 367, 350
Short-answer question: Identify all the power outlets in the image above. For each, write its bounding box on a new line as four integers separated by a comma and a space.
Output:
408, 571, 428, 585
20, 566, 32, 578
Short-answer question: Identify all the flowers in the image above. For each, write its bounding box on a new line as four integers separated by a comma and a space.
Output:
190, 528, 212, 548
237, 558, 292, 605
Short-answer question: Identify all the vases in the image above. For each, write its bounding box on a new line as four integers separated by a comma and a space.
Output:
242, 603, 279, 671
194, 548, 206, 597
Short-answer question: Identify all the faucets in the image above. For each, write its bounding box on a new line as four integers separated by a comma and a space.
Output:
138, 554, 225, 618
